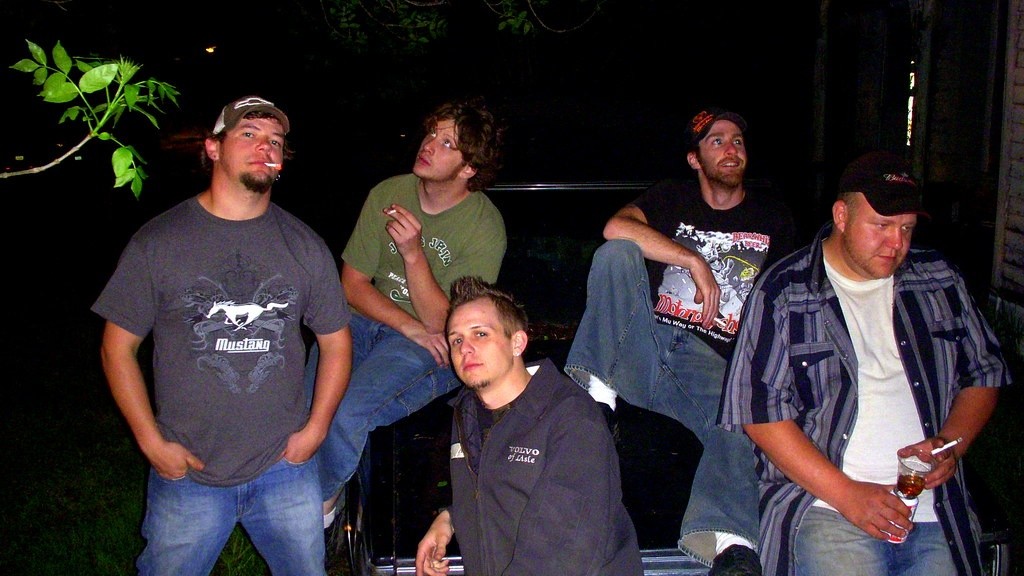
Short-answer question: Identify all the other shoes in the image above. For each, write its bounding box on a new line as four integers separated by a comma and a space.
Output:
595, 399, 621, 445
708, 544, 762, 576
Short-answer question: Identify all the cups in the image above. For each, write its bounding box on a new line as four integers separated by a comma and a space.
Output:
897, 447, 937, 499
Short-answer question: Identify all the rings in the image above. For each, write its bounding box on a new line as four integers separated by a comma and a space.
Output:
432, 560, 439, 564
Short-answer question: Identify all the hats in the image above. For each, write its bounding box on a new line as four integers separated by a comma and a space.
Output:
211, 95, 290, 136
838, 152, 932, 219
683, 106, 748, 149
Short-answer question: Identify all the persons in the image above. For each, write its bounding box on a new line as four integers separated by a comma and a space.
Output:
715, 152, 1015, 576
416, 277, 644, 576
91, 95, 354, 575
566, 109, 798, 576
303, 100, 509, 565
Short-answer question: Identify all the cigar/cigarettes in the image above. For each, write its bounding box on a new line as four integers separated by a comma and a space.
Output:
930, 436, 963, 456
714, 317, 727, 329
387, 209, 397, 215
264, 162, 282, 170
